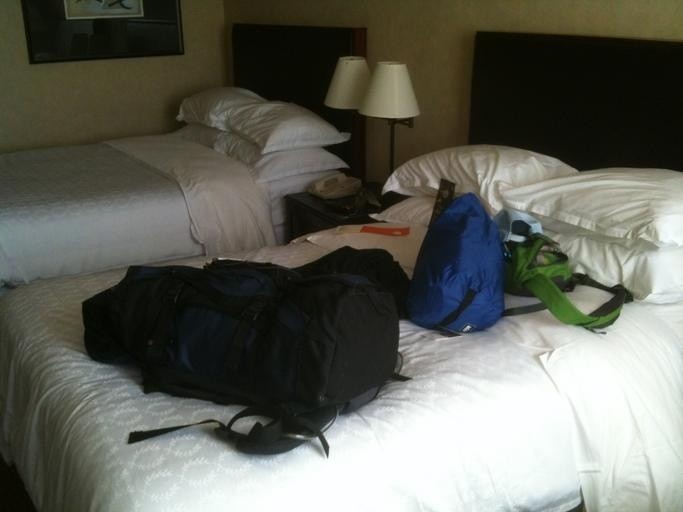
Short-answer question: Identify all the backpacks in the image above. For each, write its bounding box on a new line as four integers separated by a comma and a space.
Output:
82, 245, 409, 453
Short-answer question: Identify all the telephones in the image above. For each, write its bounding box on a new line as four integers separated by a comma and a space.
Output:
308, 174, 362, 200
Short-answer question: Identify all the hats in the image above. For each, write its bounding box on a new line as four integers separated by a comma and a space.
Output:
494, 208, 543, 241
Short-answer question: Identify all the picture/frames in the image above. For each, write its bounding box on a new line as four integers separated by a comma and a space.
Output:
20, 0, 186, 64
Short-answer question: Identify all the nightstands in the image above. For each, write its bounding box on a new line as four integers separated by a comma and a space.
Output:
283, 182, 387, 241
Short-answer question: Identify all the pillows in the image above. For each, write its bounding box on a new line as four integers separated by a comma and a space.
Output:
175, 86, 351, 183
378, 144, 580, 216
526, 228, 681, 303
370, 193, 442, 230
493, 164, 681, 254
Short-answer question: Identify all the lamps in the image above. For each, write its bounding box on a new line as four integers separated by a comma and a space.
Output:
324, 56, 372, 174
358, 61, 421, 172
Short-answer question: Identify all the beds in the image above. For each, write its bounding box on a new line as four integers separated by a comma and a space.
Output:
1, 25, 367, 286
0, 29, 683, 512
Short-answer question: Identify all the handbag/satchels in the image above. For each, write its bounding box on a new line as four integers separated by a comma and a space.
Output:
411, 192, 504, 335
502, 233, 574, 296
